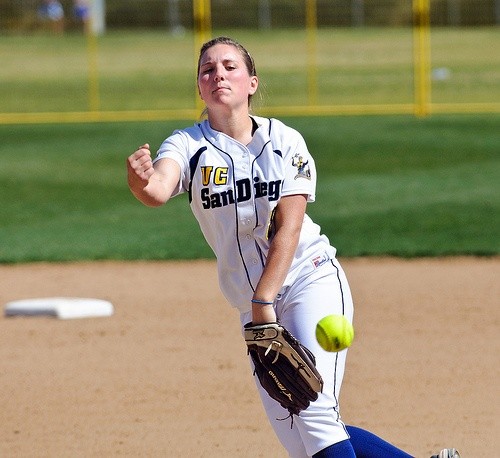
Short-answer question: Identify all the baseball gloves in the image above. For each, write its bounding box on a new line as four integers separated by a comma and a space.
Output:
242, 321, 324, 417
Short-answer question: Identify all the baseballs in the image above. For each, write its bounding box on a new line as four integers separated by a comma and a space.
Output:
314, 314, 355, 352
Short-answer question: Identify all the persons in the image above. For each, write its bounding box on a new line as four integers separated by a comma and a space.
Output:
126, 37, 462, 458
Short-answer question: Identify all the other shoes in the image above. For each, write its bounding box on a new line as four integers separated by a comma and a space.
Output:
439, 448, 461, 458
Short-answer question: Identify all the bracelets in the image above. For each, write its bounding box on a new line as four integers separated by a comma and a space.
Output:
251, 299, 274, 304
